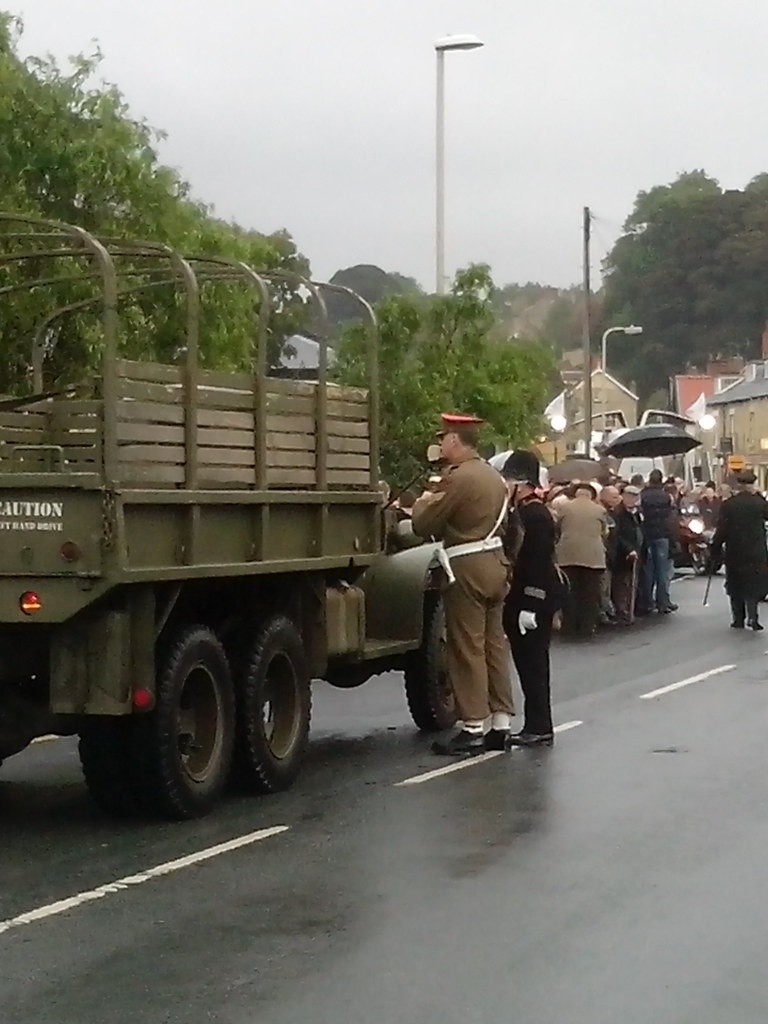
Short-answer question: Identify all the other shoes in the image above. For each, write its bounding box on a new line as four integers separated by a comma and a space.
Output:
663, 607, 672, 613
668, 604, 679, 611
731, 622, 744, 628
747, 618, 764, 631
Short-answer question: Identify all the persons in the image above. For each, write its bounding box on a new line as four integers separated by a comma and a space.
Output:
379, 413, 565, 756
532, 469, 768, 641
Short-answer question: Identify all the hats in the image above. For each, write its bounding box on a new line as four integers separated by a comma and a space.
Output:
501, 450, 545, 491
570, 483, 597, 500
436, 413, 484, 438
736, 470, 757, 484
624, 485, 641, 495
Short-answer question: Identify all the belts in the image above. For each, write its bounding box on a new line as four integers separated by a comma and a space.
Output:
444, 537, 503, 559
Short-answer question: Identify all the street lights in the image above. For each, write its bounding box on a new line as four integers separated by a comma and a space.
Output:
433, 33, 486, 299
601, 326, 644, 437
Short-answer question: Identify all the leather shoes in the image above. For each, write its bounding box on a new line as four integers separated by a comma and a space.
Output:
430, 729, 483, 756
511, 729, 553, 747
483, 729, 512, 750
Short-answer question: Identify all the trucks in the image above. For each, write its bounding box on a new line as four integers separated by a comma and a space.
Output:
0, 212, 460, 824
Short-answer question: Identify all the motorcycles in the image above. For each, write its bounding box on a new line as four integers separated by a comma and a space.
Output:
671, 492, 714, 578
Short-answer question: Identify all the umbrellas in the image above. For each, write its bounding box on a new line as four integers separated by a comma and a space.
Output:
603, 426, 703, 470
545, 459, 606, 482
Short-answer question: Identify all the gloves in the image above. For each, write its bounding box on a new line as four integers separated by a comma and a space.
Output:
518, 610, 538, 635
